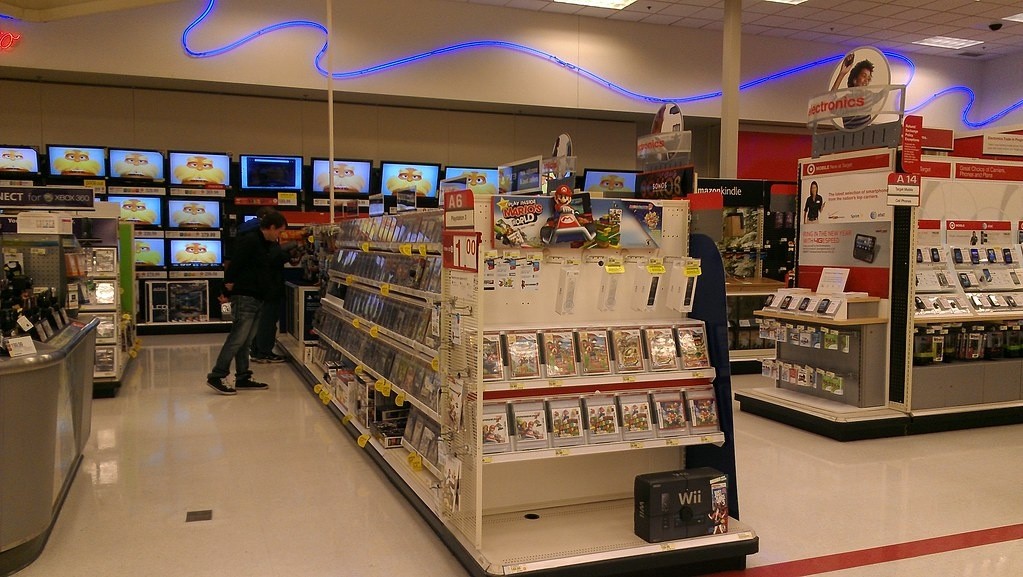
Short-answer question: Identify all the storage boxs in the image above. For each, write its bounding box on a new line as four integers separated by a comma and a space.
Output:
284, 279, 409, 449
144, 279, 210, 322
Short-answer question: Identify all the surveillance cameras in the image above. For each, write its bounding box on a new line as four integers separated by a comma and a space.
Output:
989, 23, 1002, 31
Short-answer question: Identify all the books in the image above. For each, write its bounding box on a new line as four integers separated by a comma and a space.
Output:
311, 209, 443, 465
468, 326, 720, 454
83, 248, 118, 378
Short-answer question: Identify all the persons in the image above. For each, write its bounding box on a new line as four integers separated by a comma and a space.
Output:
553, 137, 560, 156
206, 213, 288, 395
829, 54, 886, 129
804, 181, 826, 223
970, 231, 978, 245
237, 207, 297, 363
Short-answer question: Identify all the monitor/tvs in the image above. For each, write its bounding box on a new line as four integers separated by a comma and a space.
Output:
581, 169, 643, 198
0, 144, 499, 271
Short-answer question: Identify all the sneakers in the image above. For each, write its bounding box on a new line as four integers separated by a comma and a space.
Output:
265, 352, 287, 363
235, 375, 268, 390
206, 373, 237, 395
251, 353, 271, 364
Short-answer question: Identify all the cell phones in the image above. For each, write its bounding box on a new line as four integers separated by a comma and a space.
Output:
764, 294, 774, 306
853, 234, 876, 263
799, 297, 811, 311
780, 296, 792, 309
817, 299, 830, 313
915, 247, 1021, 310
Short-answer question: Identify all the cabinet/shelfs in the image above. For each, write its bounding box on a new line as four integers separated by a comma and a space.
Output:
733, 113, 1023, 442
637, 165, 795, 377
276, 190, 759, 577
725, 274, 787, 363
